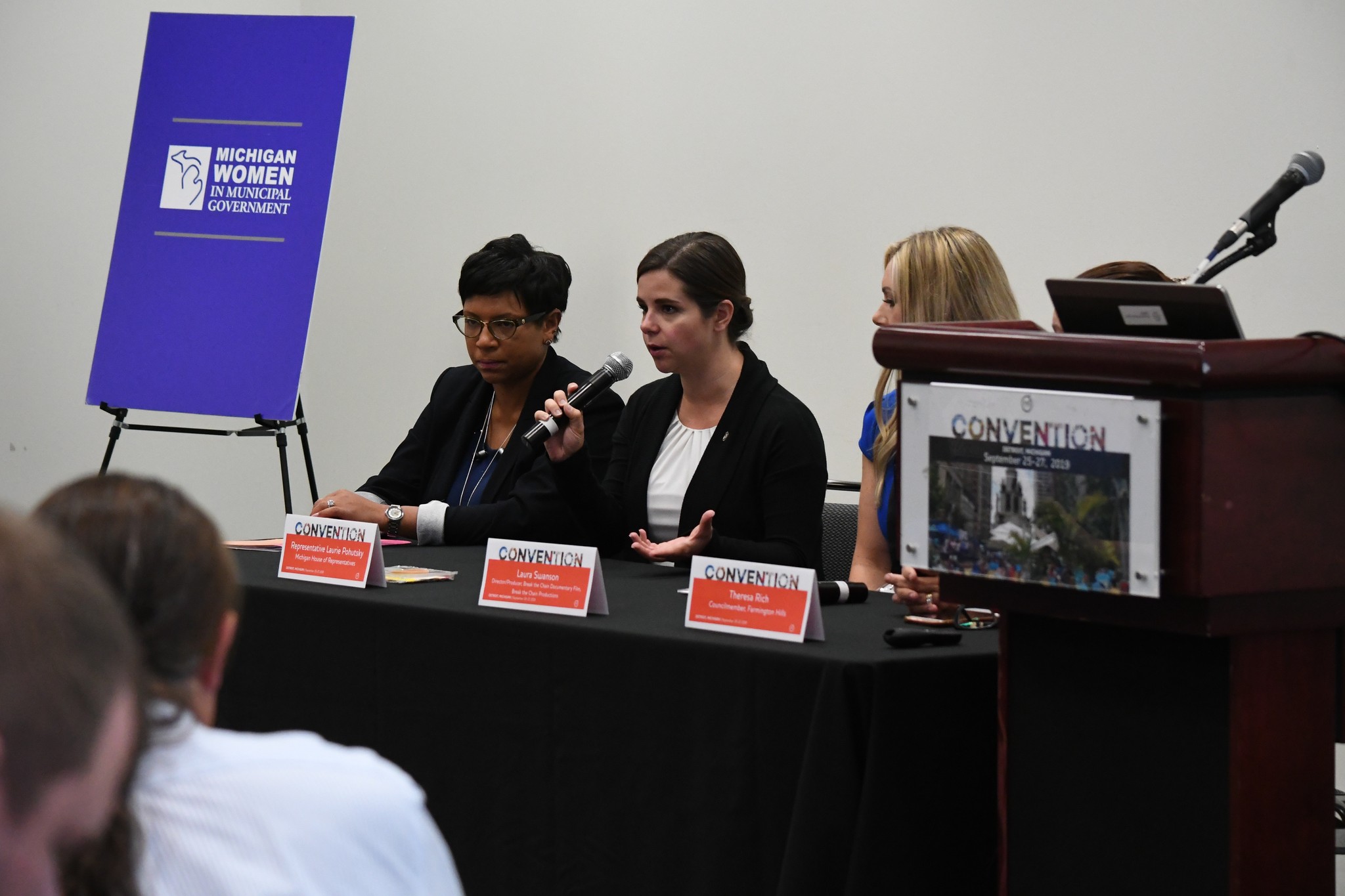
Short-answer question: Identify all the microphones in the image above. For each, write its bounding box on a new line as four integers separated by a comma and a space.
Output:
1213, 151, 1325, 249
818, 581, 868, 606
521, 351, 633, 451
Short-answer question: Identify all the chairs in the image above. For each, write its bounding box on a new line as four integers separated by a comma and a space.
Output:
821, 479, 860, 580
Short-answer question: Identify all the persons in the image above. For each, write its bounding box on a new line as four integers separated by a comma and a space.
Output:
848, 226, 1024, 594
0, 505, 147, 895
885, 261, 1183, 616
307, 234, 626, 560
28, 472, 462, 895
534, 233, 826, 582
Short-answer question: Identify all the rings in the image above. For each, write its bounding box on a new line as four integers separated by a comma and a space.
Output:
926, 593, 933, 604
327, 499, 335, 508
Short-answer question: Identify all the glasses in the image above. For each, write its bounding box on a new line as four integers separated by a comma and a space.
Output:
953, 604, 1000, 630
452, 309, 550, 340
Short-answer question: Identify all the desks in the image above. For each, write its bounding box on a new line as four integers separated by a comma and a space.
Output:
224, 540, 999, 896
871, 321, 1345, 896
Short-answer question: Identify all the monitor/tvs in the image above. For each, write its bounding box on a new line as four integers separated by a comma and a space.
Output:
1045, 277, 1244, 339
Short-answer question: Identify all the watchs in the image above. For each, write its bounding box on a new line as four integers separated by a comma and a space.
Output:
385, 504, 404, 536
